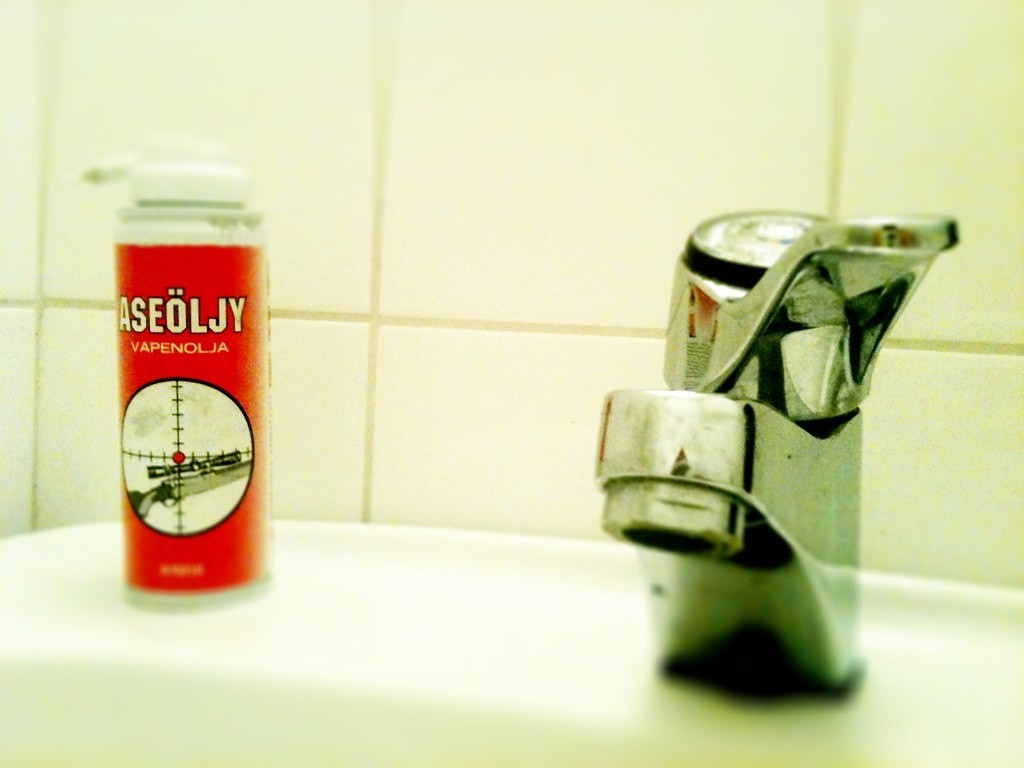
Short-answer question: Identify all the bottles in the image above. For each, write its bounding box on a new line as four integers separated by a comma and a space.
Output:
115, 168, 273, 612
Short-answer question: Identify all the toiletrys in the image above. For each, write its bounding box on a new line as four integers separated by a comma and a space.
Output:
110, 164, 271, 618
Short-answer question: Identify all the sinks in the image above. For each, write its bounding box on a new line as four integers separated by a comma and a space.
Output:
0, 514, 1022, 763
500, 512, 512, 516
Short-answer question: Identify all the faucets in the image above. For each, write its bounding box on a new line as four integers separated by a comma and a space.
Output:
598, 213, 960, 713
